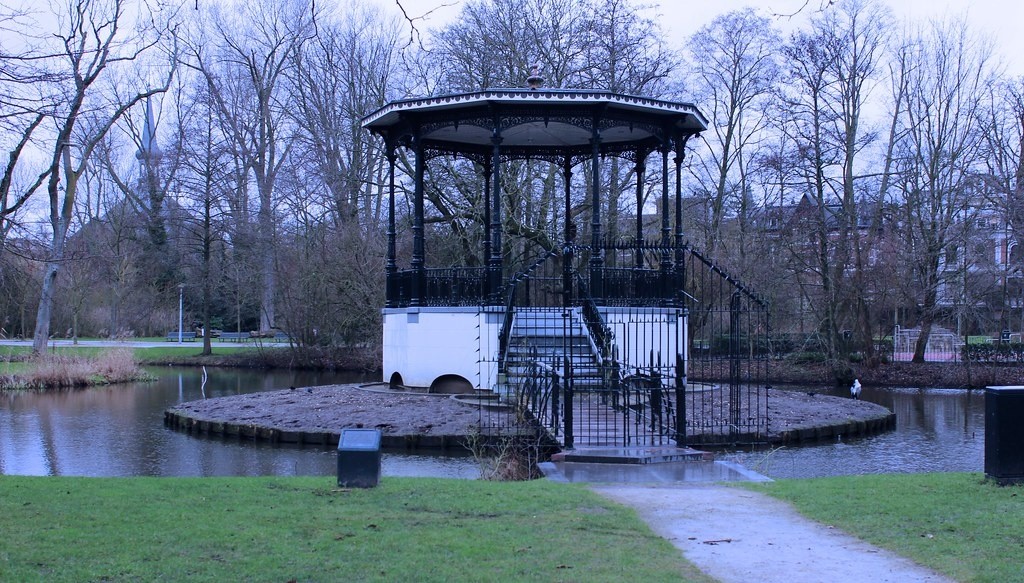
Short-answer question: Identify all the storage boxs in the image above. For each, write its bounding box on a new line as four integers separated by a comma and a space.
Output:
337, 426, 384, 488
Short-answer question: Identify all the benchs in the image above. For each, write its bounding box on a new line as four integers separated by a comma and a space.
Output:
217, 332, 250, 343
274, 331, 299, 343
166, 331, 196, 342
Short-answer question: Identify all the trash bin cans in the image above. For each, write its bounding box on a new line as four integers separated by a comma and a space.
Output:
336, 430, 382, 489
984, 386, 1024, 486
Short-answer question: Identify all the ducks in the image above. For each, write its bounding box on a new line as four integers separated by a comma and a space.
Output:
851, 379, 862, 399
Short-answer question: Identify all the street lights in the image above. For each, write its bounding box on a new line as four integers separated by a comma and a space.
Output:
179, 284, 182, 344
